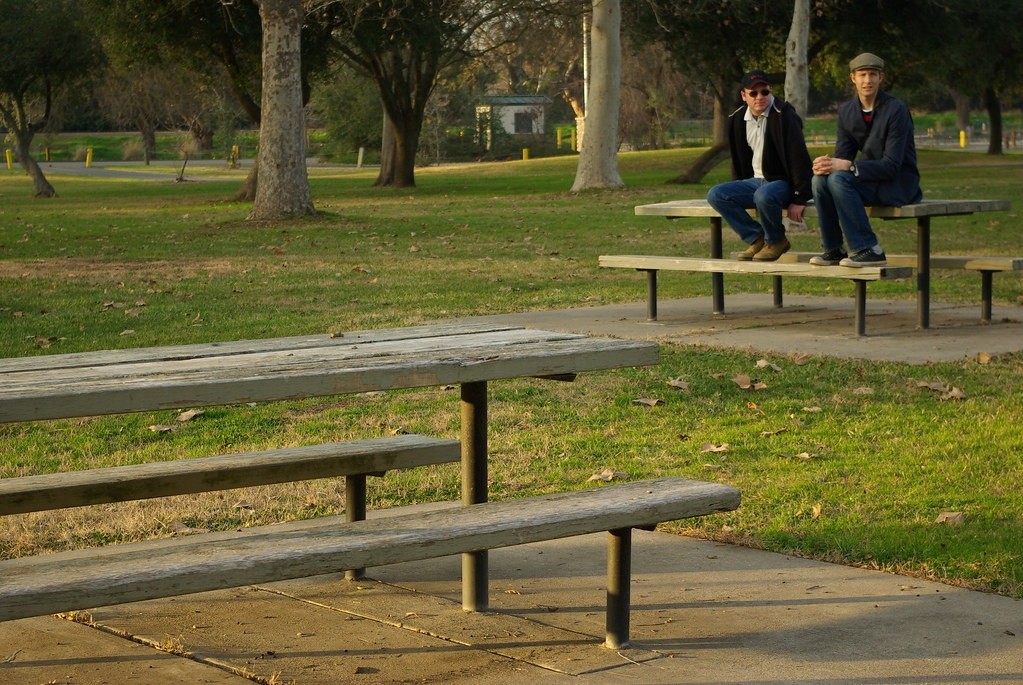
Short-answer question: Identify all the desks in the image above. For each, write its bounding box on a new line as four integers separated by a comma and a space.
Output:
639, 200, 1013, 331
0, 323, 660, 611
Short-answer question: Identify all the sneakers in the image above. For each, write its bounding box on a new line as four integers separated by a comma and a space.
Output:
736, 231, 765, 260
752, 237, 791, 262
839, 247, 887, 266
809, 248, 848, 266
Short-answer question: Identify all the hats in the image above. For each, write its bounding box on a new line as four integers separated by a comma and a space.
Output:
849, 53, 884, 72
741, 69, 771, 90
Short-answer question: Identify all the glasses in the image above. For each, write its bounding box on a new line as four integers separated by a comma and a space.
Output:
744, 89, 773, 98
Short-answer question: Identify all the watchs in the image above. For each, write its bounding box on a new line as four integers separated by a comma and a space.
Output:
849, 162, 855, 172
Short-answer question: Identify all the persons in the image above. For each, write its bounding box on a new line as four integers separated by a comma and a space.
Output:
706, 69, 814, 261
809, 53, 922, 268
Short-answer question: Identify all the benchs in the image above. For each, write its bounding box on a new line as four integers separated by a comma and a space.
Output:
599, 247, 1023, 338
0, 435, 741, 652
